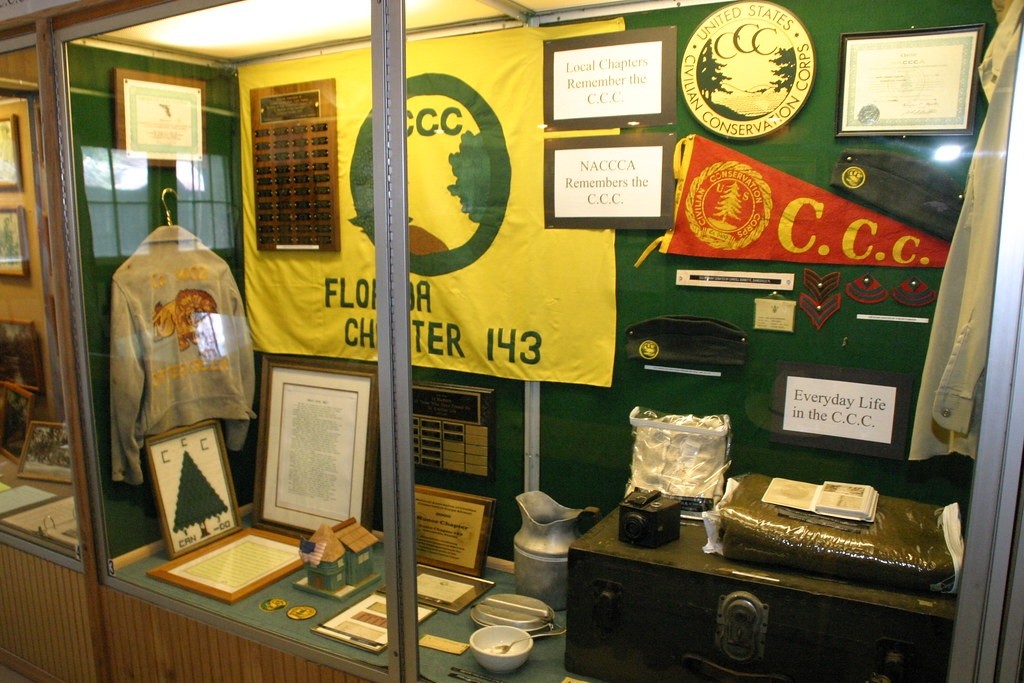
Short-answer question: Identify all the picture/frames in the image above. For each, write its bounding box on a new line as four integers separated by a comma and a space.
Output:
0, 113, 23, 193
0, 205, 31, 277
0, 317, 71, 485
834, 21, 987, 138
112, 66, 207, 170
144, 419, 242, 559
252, 353, 378, 539
145, 527, 306, 605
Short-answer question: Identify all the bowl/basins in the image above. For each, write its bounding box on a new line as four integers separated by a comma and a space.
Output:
469, 625, 533, 674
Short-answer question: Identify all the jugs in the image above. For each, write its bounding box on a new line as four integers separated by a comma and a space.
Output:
513, 490, 603, 612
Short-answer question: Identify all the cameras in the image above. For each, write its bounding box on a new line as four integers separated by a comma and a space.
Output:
619, 489, 681, 548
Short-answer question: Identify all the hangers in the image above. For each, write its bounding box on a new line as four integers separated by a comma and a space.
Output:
161, 188, 178, 227
